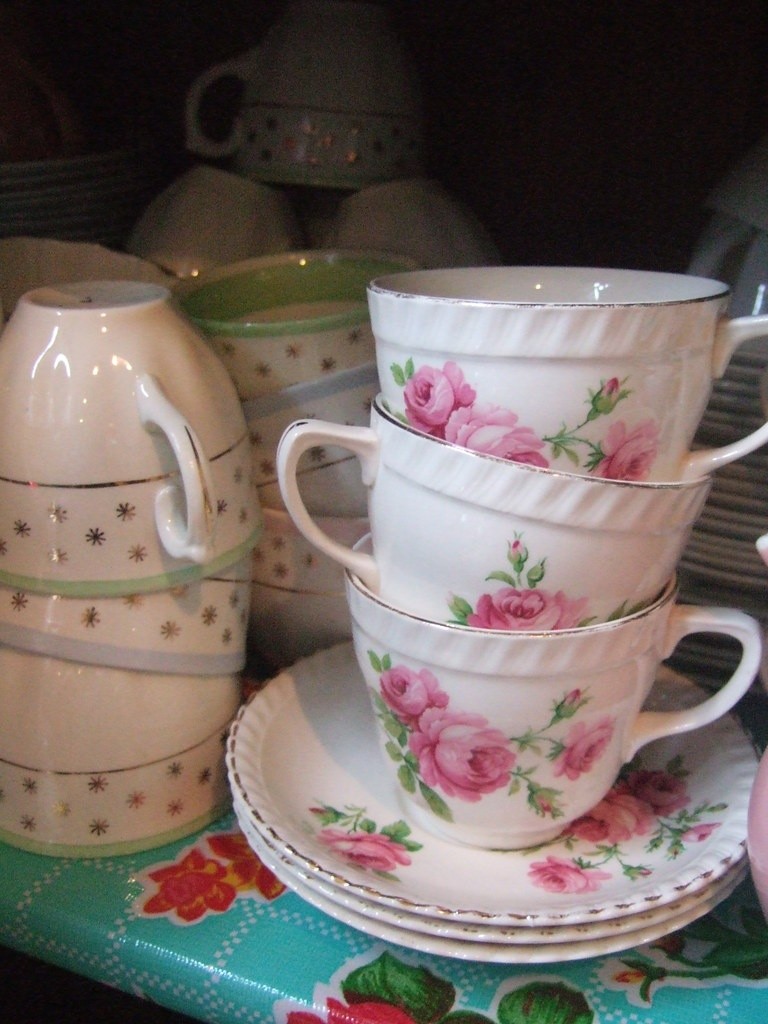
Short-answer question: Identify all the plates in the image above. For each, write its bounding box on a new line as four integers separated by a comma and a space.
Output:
0, 151, 139, 251
663, 350, 768, 696
223, 637, 761, 965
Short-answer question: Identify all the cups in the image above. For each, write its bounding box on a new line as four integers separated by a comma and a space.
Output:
125, 163, 305, 280
0, 237, 419, 859
275, 265, 768, 851
184, 0, 432, 189
684, 138, 768, 350
0, 6, 93, 161
306, 177, 504, 271
